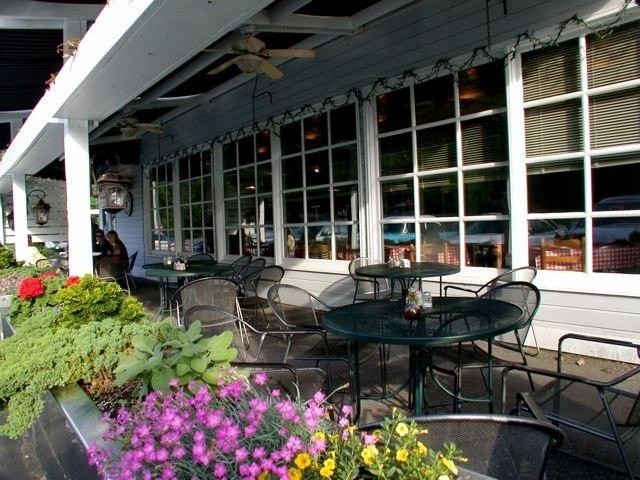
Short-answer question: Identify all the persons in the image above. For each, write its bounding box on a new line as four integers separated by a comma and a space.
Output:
154, 225, 172, 242
287, 228, 295, 258
95, 227, 109, 258
104, 229, 130, 258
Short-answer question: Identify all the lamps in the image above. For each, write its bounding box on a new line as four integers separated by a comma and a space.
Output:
25, 188, 53, 227
91, 150, 132, 217
5, 209, 14, 232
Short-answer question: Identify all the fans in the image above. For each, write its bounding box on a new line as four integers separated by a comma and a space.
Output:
114, 117, 165, 142
201, 25, 315, 82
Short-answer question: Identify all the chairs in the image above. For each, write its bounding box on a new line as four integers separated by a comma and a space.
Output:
351, 391, 566, 480
143, 251, 291, 339
348, 256, 403, 303
426, 280, 543, 412
266, 283, 383, 395
441, 265, 540, 344
36, 247, 140, 296
500, 331, 639, 480
182, 305, 333, 420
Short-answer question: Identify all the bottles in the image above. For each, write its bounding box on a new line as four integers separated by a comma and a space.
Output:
421, 292, 433, 310
404, 289, 421, 320
388, 257, 411, 268
162, 257, 190, 270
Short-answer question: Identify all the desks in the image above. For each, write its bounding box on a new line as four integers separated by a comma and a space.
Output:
317, 295, 527, 421
357, 259, 462, 293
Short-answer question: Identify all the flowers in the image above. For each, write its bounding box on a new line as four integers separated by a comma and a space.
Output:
86, 376, 470, 479
